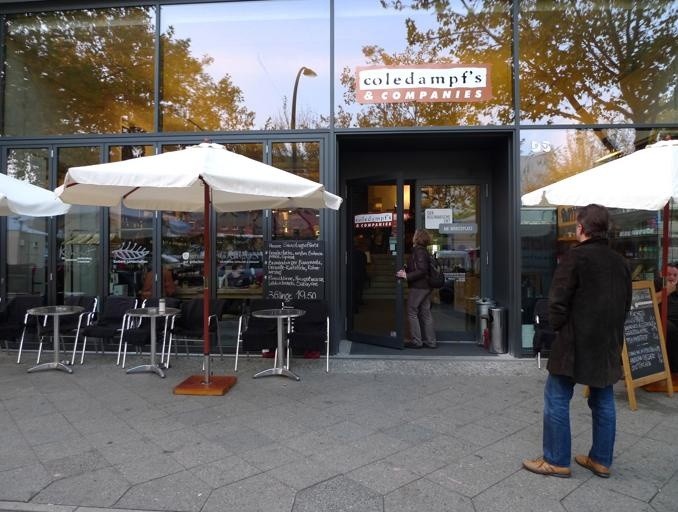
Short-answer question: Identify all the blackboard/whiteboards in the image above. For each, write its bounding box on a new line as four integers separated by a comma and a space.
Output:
265, 239, 324, 302
621, 280, 671, 389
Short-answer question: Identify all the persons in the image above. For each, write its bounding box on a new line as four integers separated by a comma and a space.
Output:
141, 260, 176, 299
396, 229, 444, 348
523, 204, 632, 478
656, 262, 677, 336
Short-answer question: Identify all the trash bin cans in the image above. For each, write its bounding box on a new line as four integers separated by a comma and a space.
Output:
488, 307, 507, 354
475, 296, 497, 346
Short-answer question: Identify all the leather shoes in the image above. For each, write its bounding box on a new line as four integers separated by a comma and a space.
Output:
575, 455, 611, 478
523, 458, 570, 477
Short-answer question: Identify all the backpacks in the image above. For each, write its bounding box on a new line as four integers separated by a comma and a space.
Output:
428, 253, 444, 288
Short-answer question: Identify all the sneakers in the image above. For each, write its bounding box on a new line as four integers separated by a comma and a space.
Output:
424, 342, 437, 348
405, 341, 421, 348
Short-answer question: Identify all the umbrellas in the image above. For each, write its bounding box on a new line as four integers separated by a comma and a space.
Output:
0, 171, 72, 218
60, 139, 344, 395
520, 140, 677, 213
